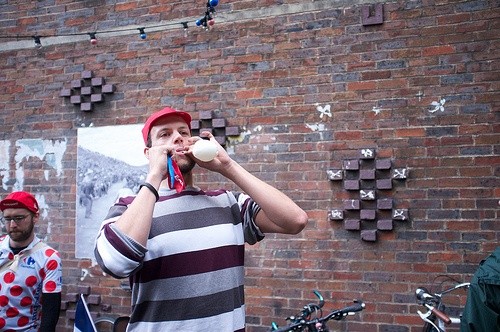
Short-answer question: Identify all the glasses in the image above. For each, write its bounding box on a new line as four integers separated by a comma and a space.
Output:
1, 213, 33, 224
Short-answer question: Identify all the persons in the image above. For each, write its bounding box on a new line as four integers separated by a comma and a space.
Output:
93, 108, 310, 332
77, 145, 146, 219
0, 191, 62, 332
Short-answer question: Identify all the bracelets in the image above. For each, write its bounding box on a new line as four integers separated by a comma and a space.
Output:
138, 182, 160, 202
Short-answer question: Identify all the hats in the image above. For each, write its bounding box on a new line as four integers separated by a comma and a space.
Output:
0, 191, 40, 215
142, 106, 192, 145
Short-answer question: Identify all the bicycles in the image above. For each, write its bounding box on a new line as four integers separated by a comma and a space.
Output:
269, 274, 472, 332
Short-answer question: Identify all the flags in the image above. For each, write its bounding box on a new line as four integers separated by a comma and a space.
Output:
74, 296, 95, 332
